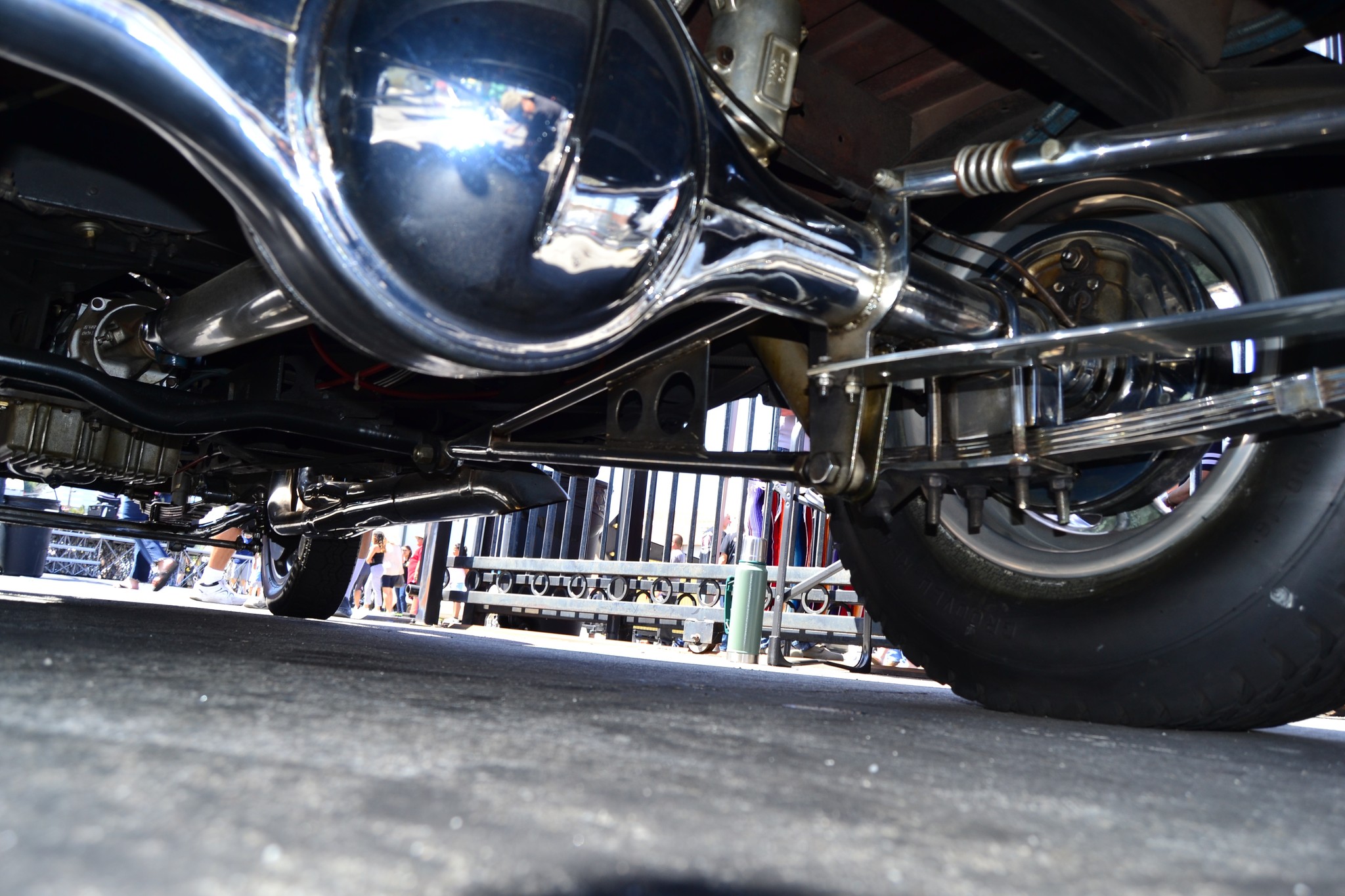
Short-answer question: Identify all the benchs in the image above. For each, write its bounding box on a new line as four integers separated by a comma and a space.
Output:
47, 526, 102, 575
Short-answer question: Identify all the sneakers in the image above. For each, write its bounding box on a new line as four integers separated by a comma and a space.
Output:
189, 575, 251, 605
243, 587, 269, 610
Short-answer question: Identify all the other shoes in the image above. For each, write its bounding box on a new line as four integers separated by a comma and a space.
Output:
333, 596, 352, 618
443, 617, 460, 623
371, 608, 381, 613
358, 606, 369, 611
395, 613, 402, 617
382, 611, 395, 616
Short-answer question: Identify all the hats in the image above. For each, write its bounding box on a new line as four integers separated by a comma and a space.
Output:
415, 532, 425, 539
401, 546, 409, 550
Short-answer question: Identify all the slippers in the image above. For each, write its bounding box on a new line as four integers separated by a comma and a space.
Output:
152, 563, 179, 592
113, 581, 127, 588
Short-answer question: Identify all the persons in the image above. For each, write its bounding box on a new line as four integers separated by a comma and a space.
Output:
353, 532, 425, 618
119, 492, 179, 592
443, 545, 470, 625
334, 530, 373, 618
655, 534, 685, 646
1162, 442, 1222, 507
190, 527, 243, 606
719, 526, 746, 652
696, 513, 732, 584
758, 637, 924, 668
231, 533, 267, 609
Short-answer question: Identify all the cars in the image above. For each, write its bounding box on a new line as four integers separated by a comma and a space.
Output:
0, 0, 1345, 729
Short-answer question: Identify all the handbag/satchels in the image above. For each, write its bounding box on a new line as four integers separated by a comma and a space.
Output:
394, 575, 405, 588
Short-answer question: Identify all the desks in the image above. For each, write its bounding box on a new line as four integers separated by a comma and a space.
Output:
90, 532, 170, 586
176, 545, 254, 594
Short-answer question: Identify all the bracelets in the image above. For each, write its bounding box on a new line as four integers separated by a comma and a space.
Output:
1167, 492, 1176, 506
